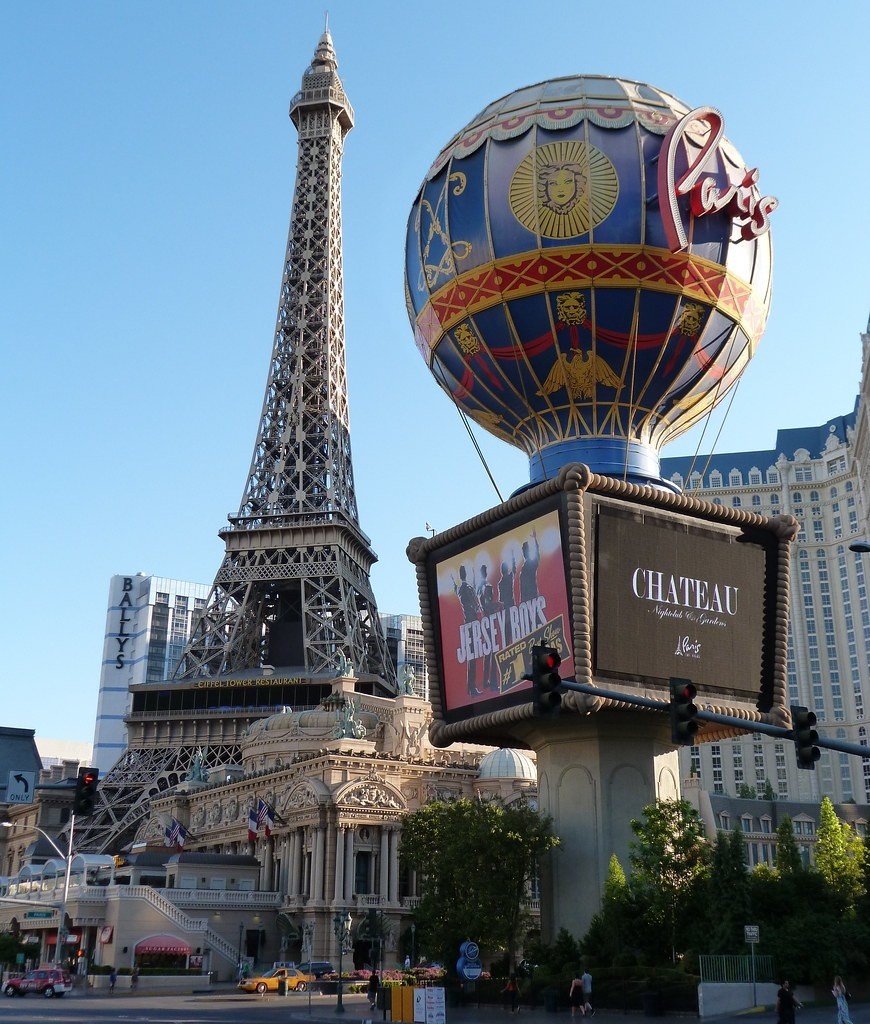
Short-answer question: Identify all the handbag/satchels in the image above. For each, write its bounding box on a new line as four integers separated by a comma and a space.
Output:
110, 982, 113, 987
845, 990, 852, 1002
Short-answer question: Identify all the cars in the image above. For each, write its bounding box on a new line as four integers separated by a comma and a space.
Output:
237, 967, 315, 992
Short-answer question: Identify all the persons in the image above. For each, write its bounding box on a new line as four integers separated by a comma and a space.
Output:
60, 957, 78, 974
775, 979, 803, 1024
26, 957, 31, 973
189, 955, 203, 968
581, 968, 596, 1017
405, 954, 410, 969
500, 975, 520, 1015
109, 968, 116, 994
570, 973, 586, 1019
130, 963, 140, 988
448, 526, 543, 697
242, 959, 249, 979
832, 975, 854, 1024
367, 970, 379, 1011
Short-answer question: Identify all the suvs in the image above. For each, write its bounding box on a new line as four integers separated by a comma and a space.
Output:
296, 961, 337, 977
2, 968, 73, 998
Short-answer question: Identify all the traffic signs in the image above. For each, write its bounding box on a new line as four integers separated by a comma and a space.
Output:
6, 769, 36, 803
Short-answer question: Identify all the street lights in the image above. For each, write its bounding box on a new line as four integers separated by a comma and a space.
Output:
1, 815, 75, 966
411, 922, 415, 967
332, 908, 353, 1014
237, 922, 245, 972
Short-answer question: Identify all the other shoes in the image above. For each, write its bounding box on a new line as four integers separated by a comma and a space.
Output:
517, 1007, 520, 1014
591, 1010, 596, 1016
509, 1012, 514, 1014
370, 1004, 376, 1009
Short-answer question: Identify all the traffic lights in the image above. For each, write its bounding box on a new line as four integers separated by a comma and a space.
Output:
532, 645, 562, 719
364, 908, 377, 934
791, 705, 820, 770
77, 949, 85, 957
668, 677, 700, 746
74, 766, 101, 817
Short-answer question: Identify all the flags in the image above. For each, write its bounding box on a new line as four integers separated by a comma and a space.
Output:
164, 819, 186, 847
248, 799, 276, 842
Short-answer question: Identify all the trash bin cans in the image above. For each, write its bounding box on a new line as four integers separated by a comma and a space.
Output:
278, 977, 288, 996
377, 987, 391, 1010
